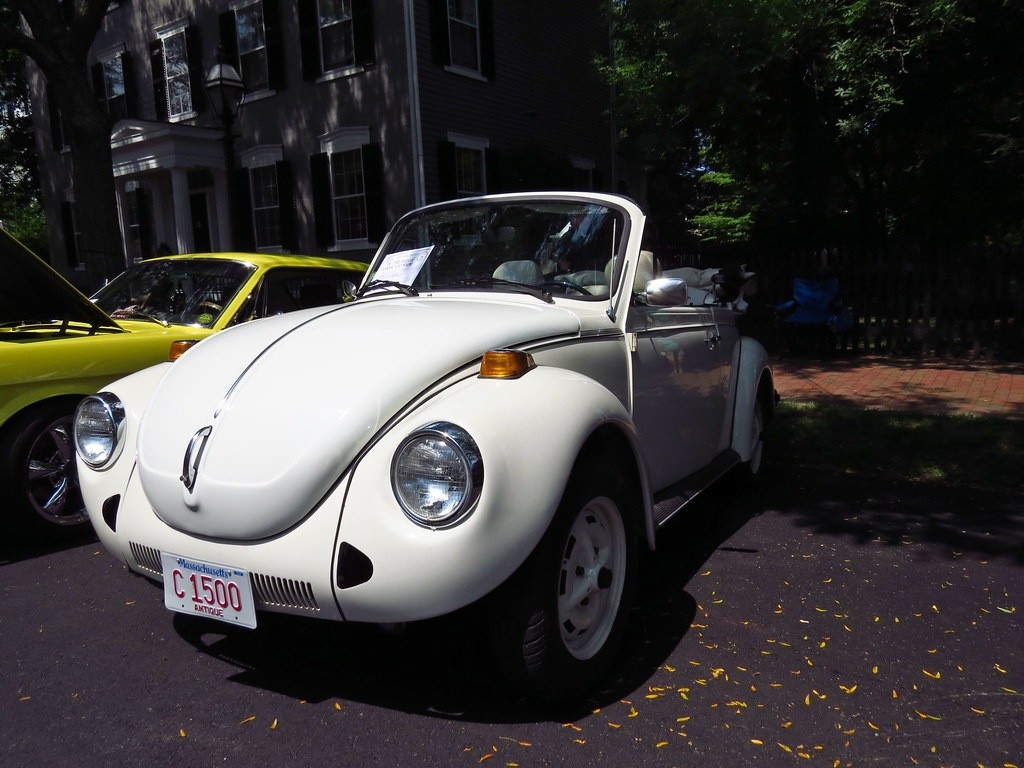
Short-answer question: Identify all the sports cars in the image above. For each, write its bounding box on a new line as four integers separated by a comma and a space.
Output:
0, 221, 380, 545
73, 188, 782, 712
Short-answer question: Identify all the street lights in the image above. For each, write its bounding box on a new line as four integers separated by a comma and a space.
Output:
201, 44, 249, 252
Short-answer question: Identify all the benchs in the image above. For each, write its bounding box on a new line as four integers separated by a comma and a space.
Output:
555, 268, 714, 305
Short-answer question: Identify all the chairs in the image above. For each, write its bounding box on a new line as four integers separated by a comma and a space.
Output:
774, 276, 850, 361
604, 250, 660, 294
492, 260, 544, 292
300, 284, 328, 308
256, 280, 298, 317
